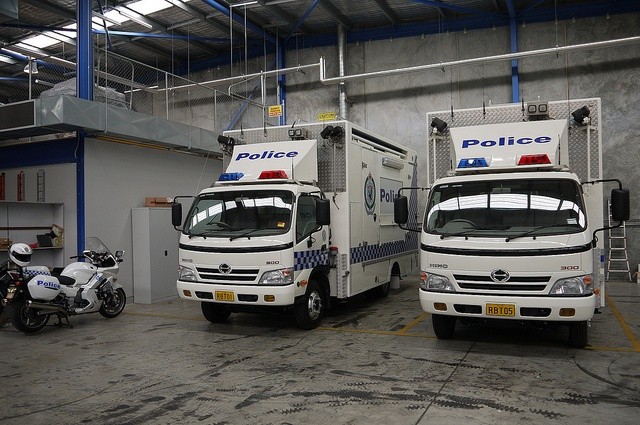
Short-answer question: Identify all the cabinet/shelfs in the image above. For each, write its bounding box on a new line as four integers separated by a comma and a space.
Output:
0, 200, 65, 271
132, 207, 180, 305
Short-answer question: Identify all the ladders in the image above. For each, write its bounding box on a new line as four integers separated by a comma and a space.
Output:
605, 198, 632, 283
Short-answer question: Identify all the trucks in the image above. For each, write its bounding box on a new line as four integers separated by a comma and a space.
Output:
393, 96, 630, 349
171, 119, 419, 331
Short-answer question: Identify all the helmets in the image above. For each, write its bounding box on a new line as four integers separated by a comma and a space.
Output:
9, 243, 33, 267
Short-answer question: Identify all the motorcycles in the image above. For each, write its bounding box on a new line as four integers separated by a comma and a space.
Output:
0, 236, 127, 335
